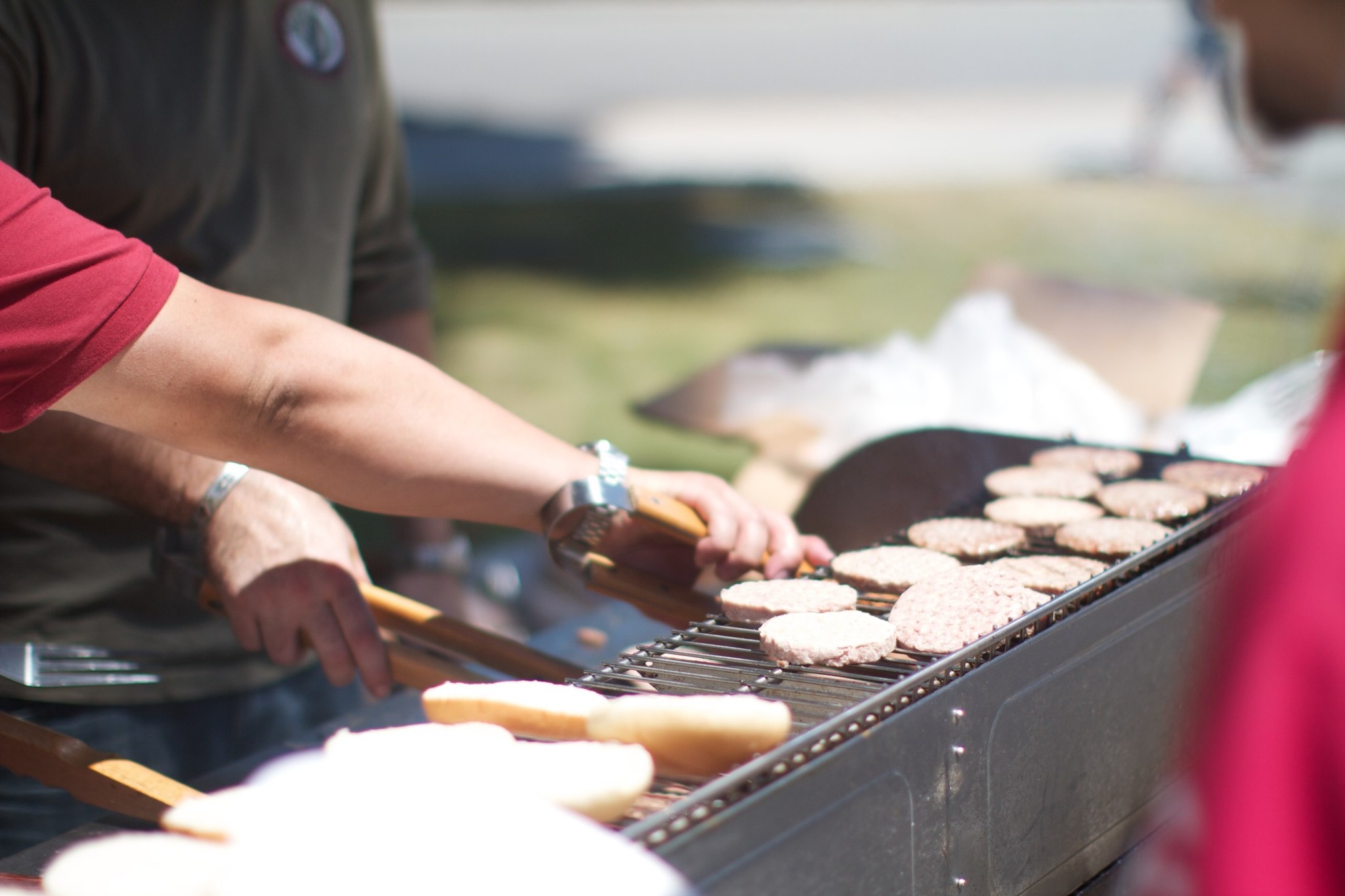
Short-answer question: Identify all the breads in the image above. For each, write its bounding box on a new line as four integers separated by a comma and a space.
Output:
421, 680, 790, 824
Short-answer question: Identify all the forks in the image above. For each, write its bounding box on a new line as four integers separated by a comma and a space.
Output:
0, 641, 160, 688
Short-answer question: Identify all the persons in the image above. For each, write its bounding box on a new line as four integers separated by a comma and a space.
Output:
0, 0, 441, 700
1158, 1, 1345, 894
0, 151, 833, 619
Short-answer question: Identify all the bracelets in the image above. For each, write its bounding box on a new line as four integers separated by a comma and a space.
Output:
194, 459, 251, 530
578, 437, 631, 544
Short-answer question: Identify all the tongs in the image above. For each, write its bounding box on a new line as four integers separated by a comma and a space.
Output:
538, 479, 822, 626
151, 527, 585, 694
